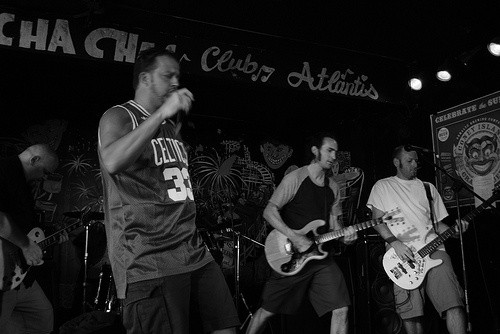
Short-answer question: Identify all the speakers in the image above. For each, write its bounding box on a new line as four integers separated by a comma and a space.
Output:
353, 234, 407, 334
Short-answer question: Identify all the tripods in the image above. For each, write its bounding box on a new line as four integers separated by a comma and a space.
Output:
230, 227, 264, 330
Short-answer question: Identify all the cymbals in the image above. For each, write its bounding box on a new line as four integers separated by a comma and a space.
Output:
62, 210, 104, 220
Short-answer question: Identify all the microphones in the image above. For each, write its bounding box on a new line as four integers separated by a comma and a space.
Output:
176, 110, 185, 128
217, 228, 231, 235
404, 144, 429, 152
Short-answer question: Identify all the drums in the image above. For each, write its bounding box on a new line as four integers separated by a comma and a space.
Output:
89, 263, 122, 316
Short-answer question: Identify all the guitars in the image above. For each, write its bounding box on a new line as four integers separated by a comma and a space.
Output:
264, 207, 406, 275
0, 220, 84, 291
382, 186, 500, 291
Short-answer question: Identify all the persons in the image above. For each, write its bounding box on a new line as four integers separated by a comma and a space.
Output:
96, 48, 241, 334
0, 144, 69, 334
245, 136, 359, 334
366, 143, 469, 334
